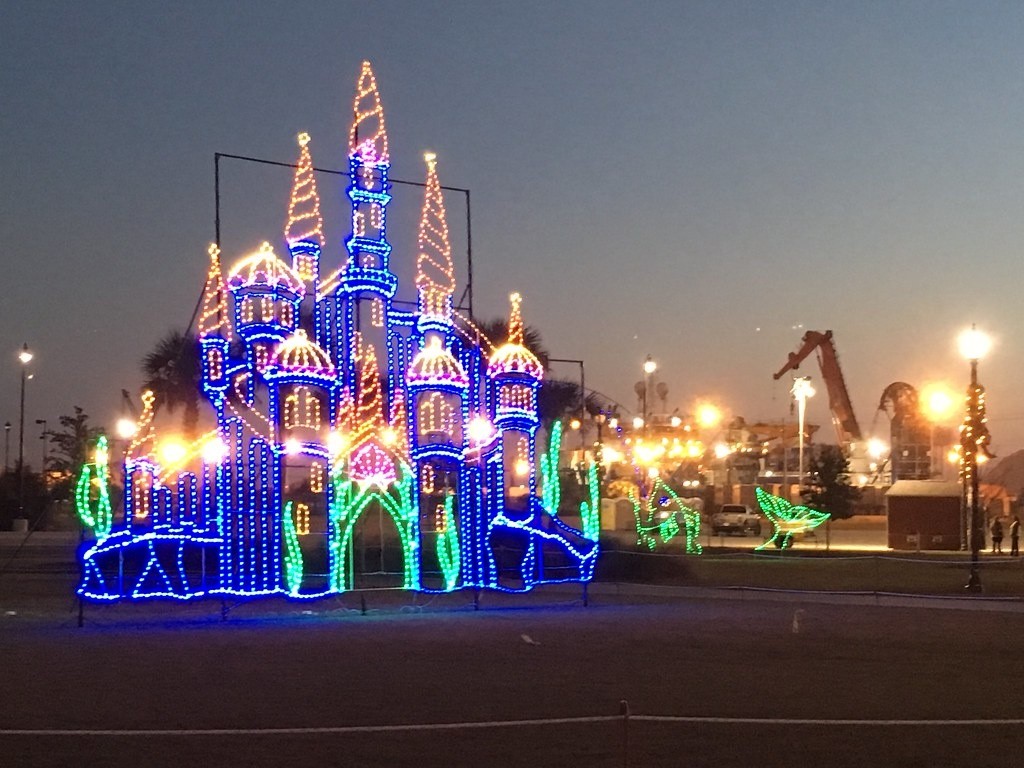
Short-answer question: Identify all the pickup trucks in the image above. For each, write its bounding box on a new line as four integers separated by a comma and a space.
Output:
710, 503, 762, 538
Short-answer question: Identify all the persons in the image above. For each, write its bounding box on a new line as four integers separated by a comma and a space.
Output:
1009, 516, 1021, 556
990, 516, 1004, 553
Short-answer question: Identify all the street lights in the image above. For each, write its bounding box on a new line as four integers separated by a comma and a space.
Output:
792, 376, 818, 489
4, 420, 12, 477
17, 341, 35, 519
958, 322, 984, 594
642, 352, 658, 427
35, 418, 47, 476
